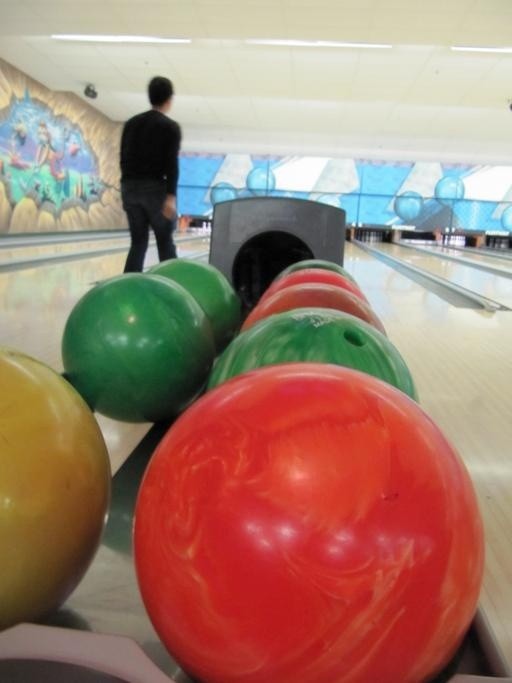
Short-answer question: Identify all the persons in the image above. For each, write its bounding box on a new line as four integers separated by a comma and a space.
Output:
121, 78, 181, 274
